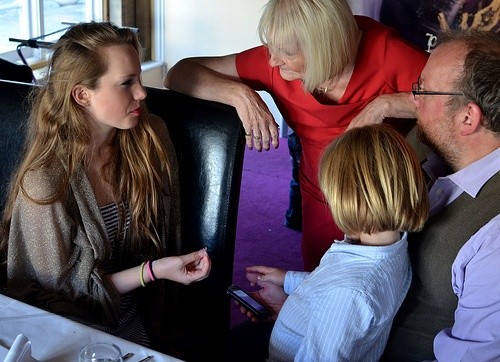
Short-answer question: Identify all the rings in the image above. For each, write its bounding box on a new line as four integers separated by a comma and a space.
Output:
253, 136, 261, 140
245, 133, 253, 137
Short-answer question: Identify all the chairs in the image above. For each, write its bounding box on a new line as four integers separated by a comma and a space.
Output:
0, 57, 36, 84
139, 86, 245, 362
0, 79, 48, 233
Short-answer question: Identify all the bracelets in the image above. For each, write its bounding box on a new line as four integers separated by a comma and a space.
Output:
139, 261, 148, 287
146, 260, 156, 283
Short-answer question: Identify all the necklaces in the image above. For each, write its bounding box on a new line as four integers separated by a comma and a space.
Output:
313, 75, 329, 101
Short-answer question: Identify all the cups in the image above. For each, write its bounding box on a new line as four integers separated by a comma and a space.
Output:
79, 341, 124, 362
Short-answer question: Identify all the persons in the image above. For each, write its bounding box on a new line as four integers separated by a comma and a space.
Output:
163, 0, 430, 272
0, 23, 212, 347
233, 29, 500, 362
246, 124, 429, 362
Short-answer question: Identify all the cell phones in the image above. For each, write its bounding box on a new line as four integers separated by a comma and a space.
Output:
227, 285, 271, 318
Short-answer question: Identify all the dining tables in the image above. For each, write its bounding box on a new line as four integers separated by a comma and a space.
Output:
0, 290, 182, 362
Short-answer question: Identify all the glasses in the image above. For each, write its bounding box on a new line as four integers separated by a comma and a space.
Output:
411, 75, 462, 99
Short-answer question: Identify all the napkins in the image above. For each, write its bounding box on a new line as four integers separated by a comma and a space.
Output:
3, 334, 32, 362
